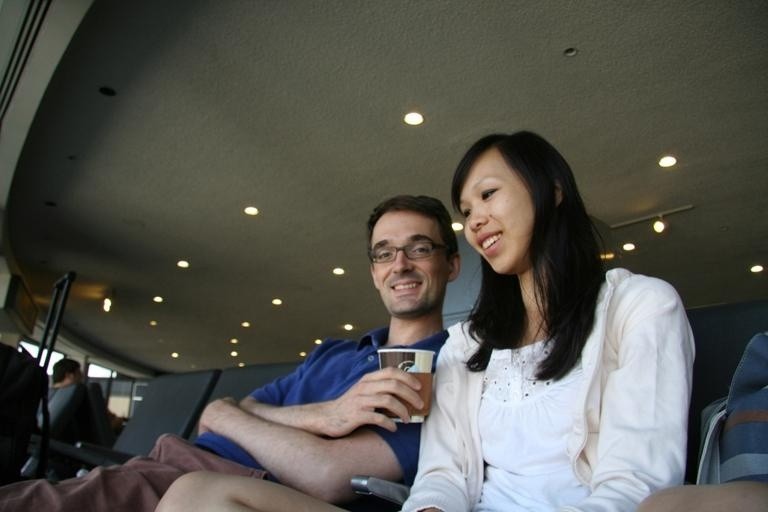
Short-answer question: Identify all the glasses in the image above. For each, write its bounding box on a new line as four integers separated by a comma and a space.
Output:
369, 241, 447, 263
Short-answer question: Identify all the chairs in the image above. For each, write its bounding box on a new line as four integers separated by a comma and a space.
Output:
19, 358, 303, 481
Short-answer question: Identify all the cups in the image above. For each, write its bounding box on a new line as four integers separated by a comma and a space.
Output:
377, 347, 437, 424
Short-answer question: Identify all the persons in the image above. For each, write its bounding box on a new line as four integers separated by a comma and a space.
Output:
38, 358, 124, 432
0, 194, 460, 511
153, 130, 767, 512
